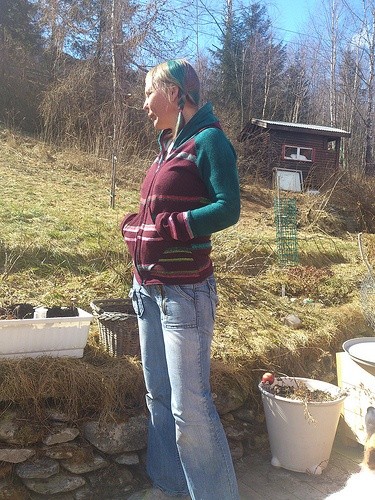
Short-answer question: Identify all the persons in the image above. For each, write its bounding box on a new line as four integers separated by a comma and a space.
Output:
121, 58, 241, 500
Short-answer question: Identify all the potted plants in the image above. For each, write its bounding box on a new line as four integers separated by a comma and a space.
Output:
257, 372, 350, 475
0, 306, 95, 359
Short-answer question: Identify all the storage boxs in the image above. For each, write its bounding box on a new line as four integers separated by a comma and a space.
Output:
90, 298, 140, 358
334, 351, 375, 450
